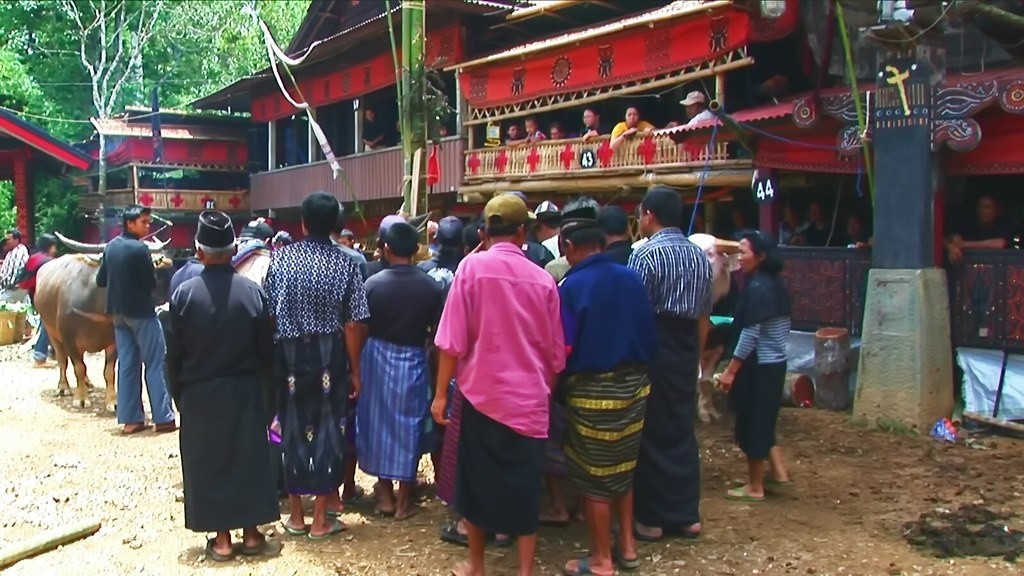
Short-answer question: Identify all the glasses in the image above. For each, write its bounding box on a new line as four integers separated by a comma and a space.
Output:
581, 115, 598, 120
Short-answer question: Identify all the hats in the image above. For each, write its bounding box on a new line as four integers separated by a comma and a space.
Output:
533, 199, 559, 215
435, 215, 463, 245
239, 221, 279, 240
484, 194, 538, 230
378, 213, 408, 245
195, 209, 234, 247
679, 91, 707, 106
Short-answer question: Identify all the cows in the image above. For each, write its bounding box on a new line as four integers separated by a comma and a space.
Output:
34, 231, 172, 413
686, 232, 741, 424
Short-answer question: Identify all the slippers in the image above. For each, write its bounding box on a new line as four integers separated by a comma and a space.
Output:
722, 487, 768, 502
180, 357, 699, 574
762, 474, 795, 488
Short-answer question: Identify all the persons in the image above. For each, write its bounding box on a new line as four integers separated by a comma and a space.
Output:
713, 228, 793, 500
20, 232, 59, 369
555, 214, 656, 575
94, 171, 251, 199
429, 193, 570, 576
162, 73, 1011, 528
165, 210, 282, 563
354, 221, 450, 523
261, 190, 370, 542
0, 231, 32, 343
626, 185, 713, 543
96, 204, 177, 435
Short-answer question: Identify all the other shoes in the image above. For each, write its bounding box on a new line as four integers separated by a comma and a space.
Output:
122, 414, 150, 434
32, 362, 57, 368
156, 418, 180, 433
